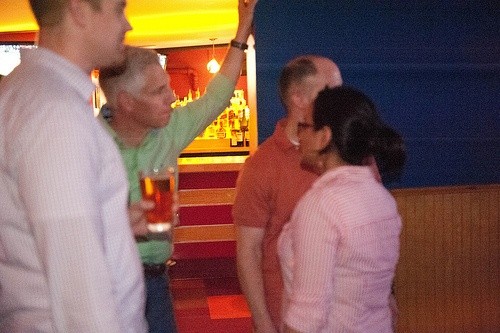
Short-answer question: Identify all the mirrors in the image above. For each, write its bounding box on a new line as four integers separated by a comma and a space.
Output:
154, 45, 249, 151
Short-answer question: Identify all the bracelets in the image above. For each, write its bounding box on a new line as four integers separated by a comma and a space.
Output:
228, 39, 250, 50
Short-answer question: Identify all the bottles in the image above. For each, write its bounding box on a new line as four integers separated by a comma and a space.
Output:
170, 87, 249, 139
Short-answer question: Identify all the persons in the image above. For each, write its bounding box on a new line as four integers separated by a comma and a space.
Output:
267, 83, 405, 333
92, 0, 258, 332
229, 52, 382, 332
0, 1, 137, 330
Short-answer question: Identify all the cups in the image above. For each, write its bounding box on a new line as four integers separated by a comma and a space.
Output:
238, 108, 250, 147
230, 118, 241, 147
137, 165, 178, 240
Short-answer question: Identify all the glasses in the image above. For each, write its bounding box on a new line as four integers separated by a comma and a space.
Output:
297, 121, 314, 136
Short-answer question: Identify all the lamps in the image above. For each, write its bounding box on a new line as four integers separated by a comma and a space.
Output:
207, 38, 220, 73
